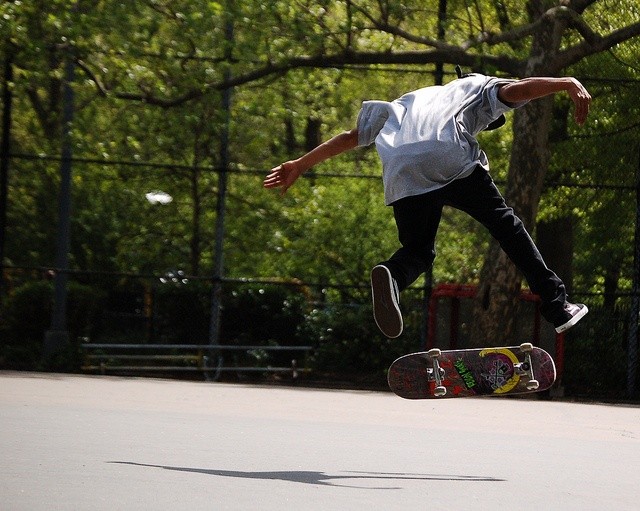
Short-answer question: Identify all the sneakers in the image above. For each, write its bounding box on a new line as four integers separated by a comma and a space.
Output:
370, 265, 404, 339
554, 303, 589, 334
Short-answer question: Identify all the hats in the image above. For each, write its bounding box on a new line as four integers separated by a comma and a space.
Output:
455, 64, 506, 130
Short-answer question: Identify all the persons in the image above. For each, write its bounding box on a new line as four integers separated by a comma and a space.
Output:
262, 63, 593, 340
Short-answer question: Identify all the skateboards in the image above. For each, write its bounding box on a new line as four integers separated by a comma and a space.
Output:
386, 343, 557, 399
